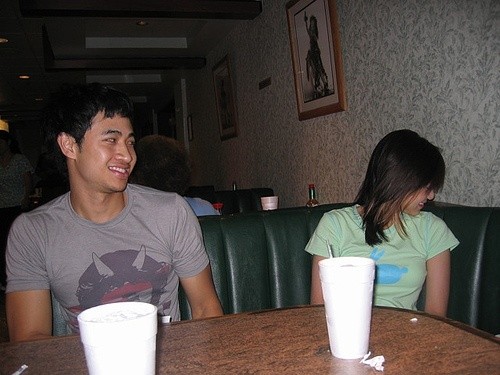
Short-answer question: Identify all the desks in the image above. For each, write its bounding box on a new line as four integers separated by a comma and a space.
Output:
0, 306, 500, 375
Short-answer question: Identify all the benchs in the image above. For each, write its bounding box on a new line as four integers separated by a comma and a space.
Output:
177, 201, 500, 340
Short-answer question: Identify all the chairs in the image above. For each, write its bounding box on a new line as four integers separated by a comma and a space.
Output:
183, 185, 278, 217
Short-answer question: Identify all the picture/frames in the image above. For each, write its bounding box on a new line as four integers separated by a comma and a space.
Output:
211, 55, 239, 141
285, 0, 348, 121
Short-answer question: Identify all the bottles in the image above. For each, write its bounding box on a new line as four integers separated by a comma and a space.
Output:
213, 202, 223, 215
231, 181, 237, 190
306, 184, 320, 207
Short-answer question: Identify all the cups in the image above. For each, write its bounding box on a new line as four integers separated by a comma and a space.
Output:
261, 196, 278, 210
318, 256, 375, 359
76, 300, 158, 375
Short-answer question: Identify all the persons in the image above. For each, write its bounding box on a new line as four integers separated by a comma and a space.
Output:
304, 129, 460, 316
130, 135, 220, 215
0, 134, 33, 291
5, 83, 223, 343
